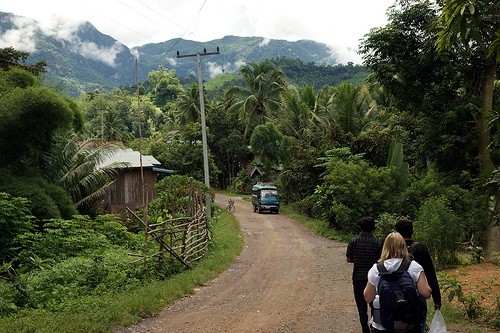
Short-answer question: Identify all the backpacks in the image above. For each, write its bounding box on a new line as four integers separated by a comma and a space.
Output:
368, 257, 428, 333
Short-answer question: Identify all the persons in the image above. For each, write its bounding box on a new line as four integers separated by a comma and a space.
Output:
395, 220, 441, 333
346, 219, 380, 333
363, 232, 432, 333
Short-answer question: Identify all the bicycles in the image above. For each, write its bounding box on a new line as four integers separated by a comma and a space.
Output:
226, 198, 235, 214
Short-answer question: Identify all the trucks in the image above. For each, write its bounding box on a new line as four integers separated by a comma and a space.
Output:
251, 183, 281, 213
